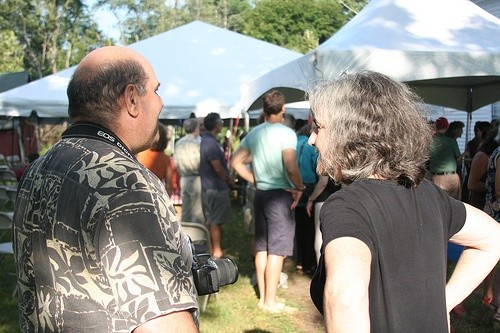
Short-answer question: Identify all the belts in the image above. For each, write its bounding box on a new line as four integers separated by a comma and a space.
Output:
437, 171, 455, 175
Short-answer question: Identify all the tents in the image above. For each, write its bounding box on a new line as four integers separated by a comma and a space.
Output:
0, 70, 65, 128
239, 0, 500, 184
0, 21, 443, 128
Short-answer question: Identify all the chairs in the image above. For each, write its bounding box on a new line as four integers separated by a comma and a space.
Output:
180, 222, 219, 311
0, 166, 19, 300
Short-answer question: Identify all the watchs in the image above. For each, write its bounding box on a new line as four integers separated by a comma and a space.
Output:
308, 196, 314, 201
298, 185, 306, 191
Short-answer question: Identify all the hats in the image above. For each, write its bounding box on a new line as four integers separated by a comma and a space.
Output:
436, 116, 448, 127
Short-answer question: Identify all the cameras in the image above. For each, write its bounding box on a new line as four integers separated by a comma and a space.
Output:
192, 257, 238, 296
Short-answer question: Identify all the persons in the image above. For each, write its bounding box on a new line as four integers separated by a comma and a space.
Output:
12, 46, 199, 333
308, 70, 500, 333
134, 113, 500, 321
232, 90, 304, 312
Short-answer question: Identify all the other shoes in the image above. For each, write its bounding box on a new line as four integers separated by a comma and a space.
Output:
264, 303, 298, 314
259, 296, 286, 308
482, 287, 495, 304
452, 304, 467, 316
